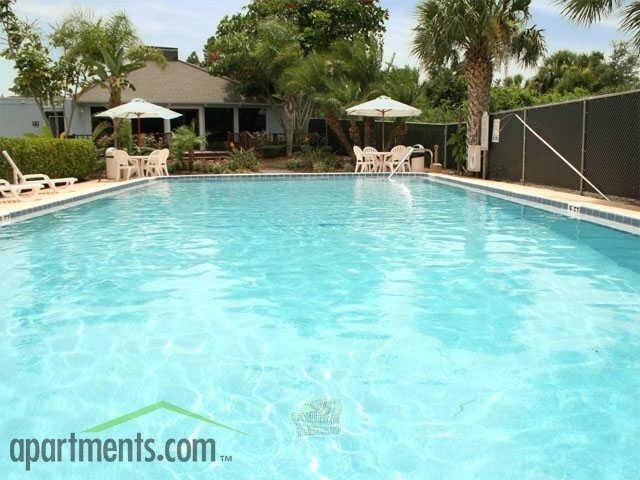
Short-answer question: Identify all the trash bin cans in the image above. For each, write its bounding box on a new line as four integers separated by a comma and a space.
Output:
411, 152, 424, 172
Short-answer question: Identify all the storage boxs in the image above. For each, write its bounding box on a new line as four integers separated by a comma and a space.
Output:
411, 156, 424, 172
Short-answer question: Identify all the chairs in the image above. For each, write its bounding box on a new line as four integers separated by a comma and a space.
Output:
353, 144, 412, 172
105, 148, 170, 180
3, 150, 78, 193
0, 179, 44, 202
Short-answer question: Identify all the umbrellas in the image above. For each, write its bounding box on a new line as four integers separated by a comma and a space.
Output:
94, 96, 182, 154
345, 94, 422, 152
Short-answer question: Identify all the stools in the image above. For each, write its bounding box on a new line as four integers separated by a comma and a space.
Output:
432, 163, 442, 173
